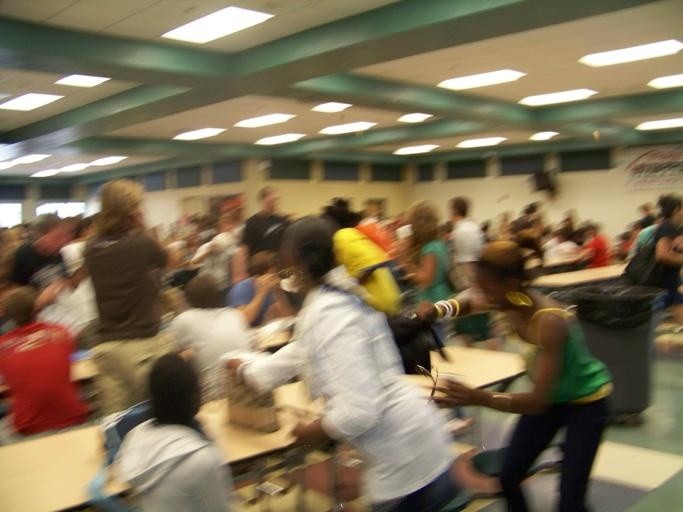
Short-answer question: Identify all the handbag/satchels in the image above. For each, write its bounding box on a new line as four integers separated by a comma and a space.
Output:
218, 369, 283, 433
387, 313, 446, 380
99, 398, 161, 461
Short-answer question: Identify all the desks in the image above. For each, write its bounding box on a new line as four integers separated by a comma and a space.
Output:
528, 263, 629, 287
1, 331, 527, 506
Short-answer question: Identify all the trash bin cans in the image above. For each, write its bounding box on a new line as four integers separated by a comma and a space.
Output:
572, 285, 668, 428
